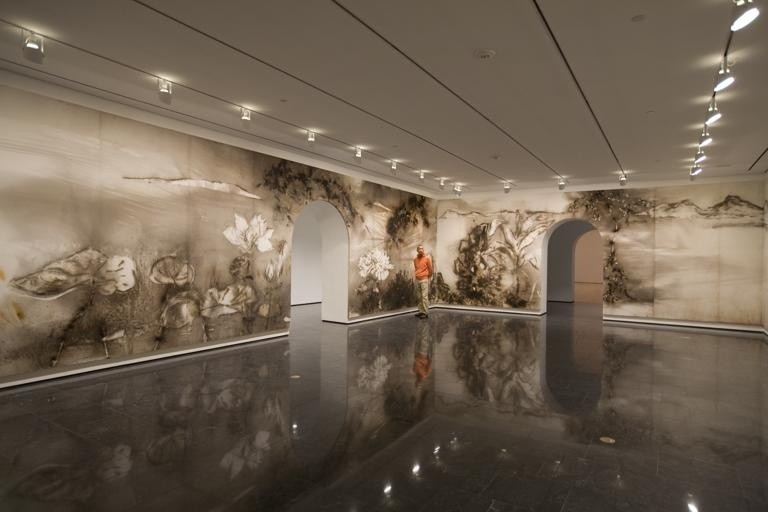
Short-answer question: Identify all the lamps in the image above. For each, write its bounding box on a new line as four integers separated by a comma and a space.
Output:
503, 182, 511, 189
22, 30, 46, 57
690, 129, 712, 176
356, 148, 362, 158
307, 132, 315, 141
241, 108, 250, 120
729, 0, 760, 32
158, 79, 173, 94
713, 56, 735, 92
392, 162, 462, 193
704, 95, 721, 126
558, 178, 566, 185
619, 174, 627, 182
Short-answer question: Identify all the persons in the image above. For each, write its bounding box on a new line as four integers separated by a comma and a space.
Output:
413, 245, 434, 319
413, 318, 437, 390
